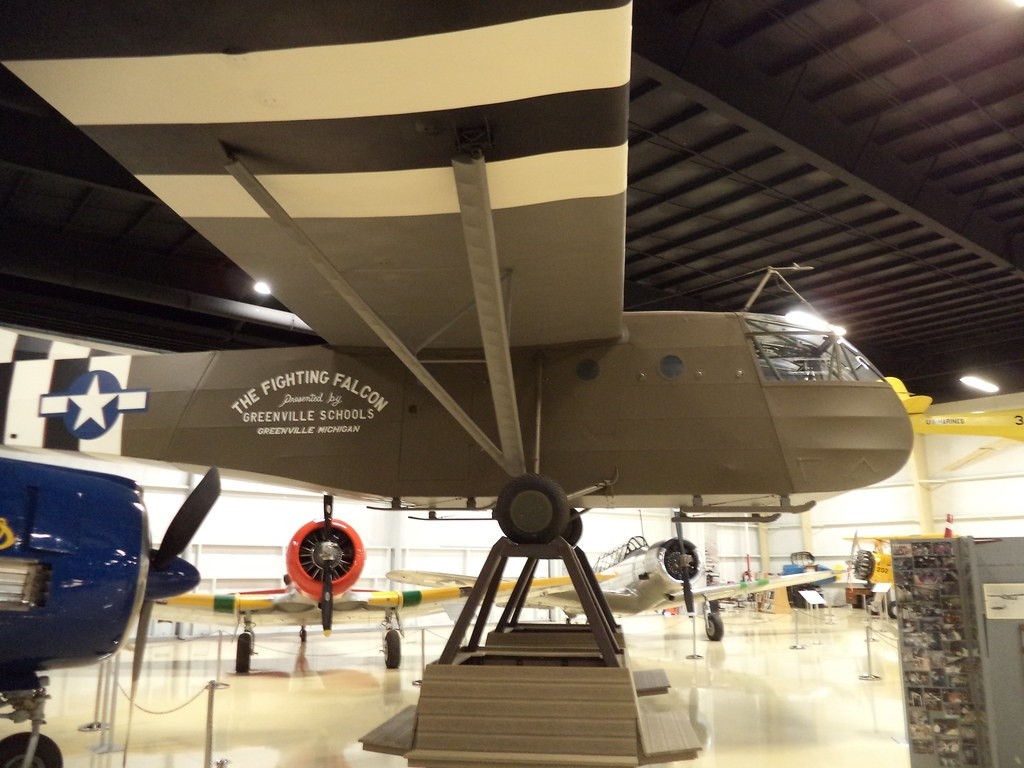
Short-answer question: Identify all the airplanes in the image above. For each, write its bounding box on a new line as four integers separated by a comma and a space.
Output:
384, 536, 847, 642
0, 456, 221, 767
145, 493, 620, 675
875, 377, 1024, 445
840, 530, 959, 592
0, 0, 912, 547
